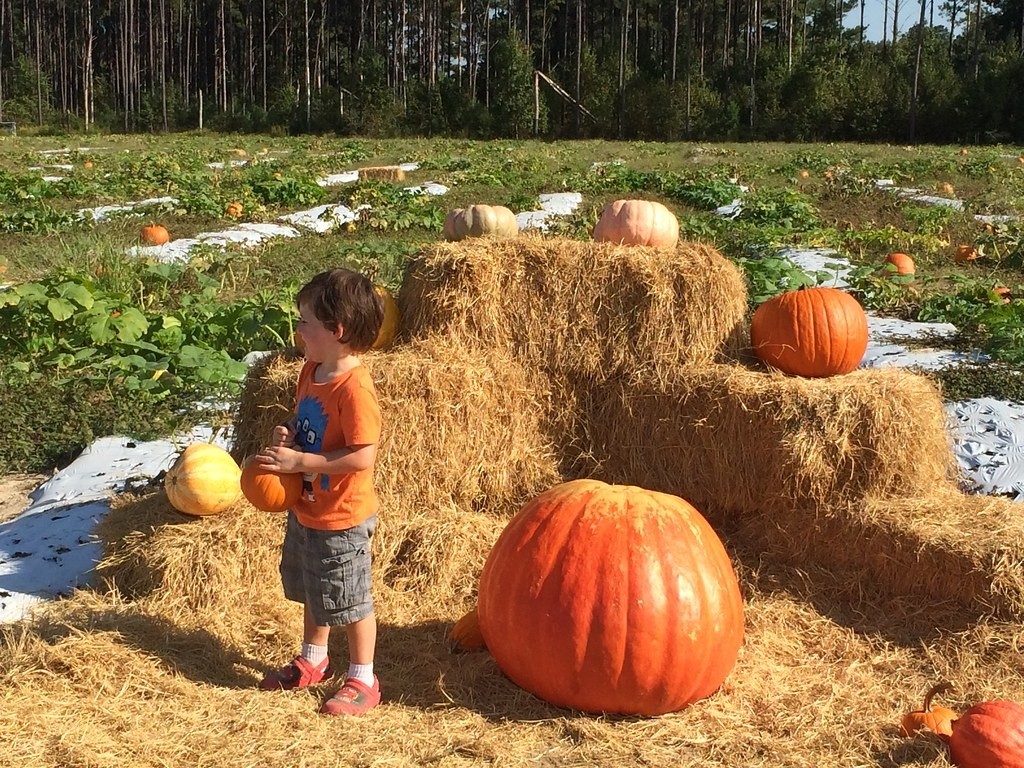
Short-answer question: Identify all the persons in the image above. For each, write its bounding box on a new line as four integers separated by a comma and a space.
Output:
254, 267, 384, 714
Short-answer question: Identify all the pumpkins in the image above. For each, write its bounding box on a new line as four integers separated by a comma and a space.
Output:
477, 478, 745, 717
900, 682, 959, 738
751, 282, 869, 378
948, 700, 1024, 768
84, 161, 401, 357
240, 459, 303, 512
592, 199, 679, 248
443, 204, 517, 242
940, 148, 1024, 305
448, 607, 485, 654
883, 253, 915, 276
165, 442, 243, 514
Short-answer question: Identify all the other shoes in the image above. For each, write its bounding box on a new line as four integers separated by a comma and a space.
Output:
320, 673, 381, 718
258, 655, 334, 691
